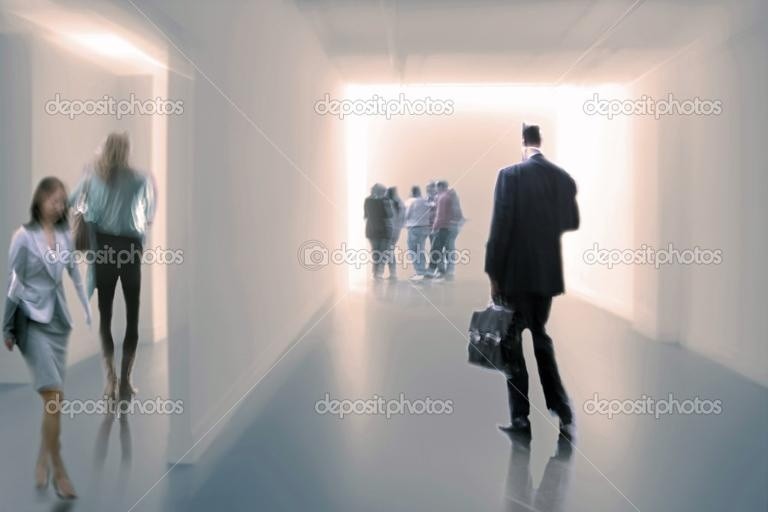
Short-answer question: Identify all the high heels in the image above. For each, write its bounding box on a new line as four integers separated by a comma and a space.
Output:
103, 375, 137, 396
34, 464, 50, 492
51, 475, 78, 500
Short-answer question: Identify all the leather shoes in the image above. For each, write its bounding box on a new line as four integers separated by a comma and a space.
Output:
557, 404, 572, 425
498, 418, 530, 432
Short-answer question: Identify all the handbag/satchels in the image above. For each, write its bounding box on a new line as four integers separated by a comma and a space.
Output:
467, 305, 517, 374
70, 212, 88, 251
